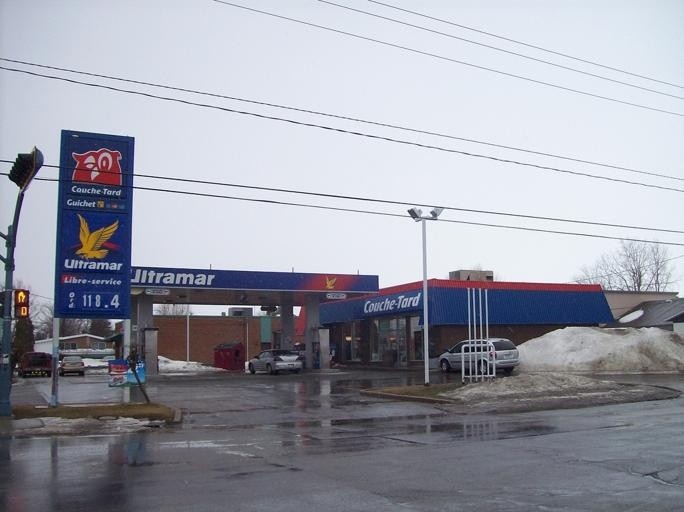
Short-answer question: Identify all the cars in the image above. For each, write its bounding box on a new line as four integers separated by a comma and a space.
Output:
58, 356, 86, 377
247, 349, 303, 374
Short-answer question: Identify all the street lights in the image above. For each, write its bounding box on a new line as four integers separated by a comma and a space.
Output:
406, 206, 445, 386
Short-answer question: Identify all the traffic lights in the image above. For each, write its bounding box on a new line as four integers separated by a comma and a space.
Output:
14, 289, 29, 319
8, 152, 33, 189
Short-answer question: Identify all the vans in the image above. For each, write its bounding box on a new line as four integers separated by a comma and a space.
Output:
439, 339, 520, 374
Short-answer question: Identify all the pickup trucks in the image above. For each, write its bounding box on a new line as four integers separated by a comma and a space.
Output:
18, 352, 52, 377
